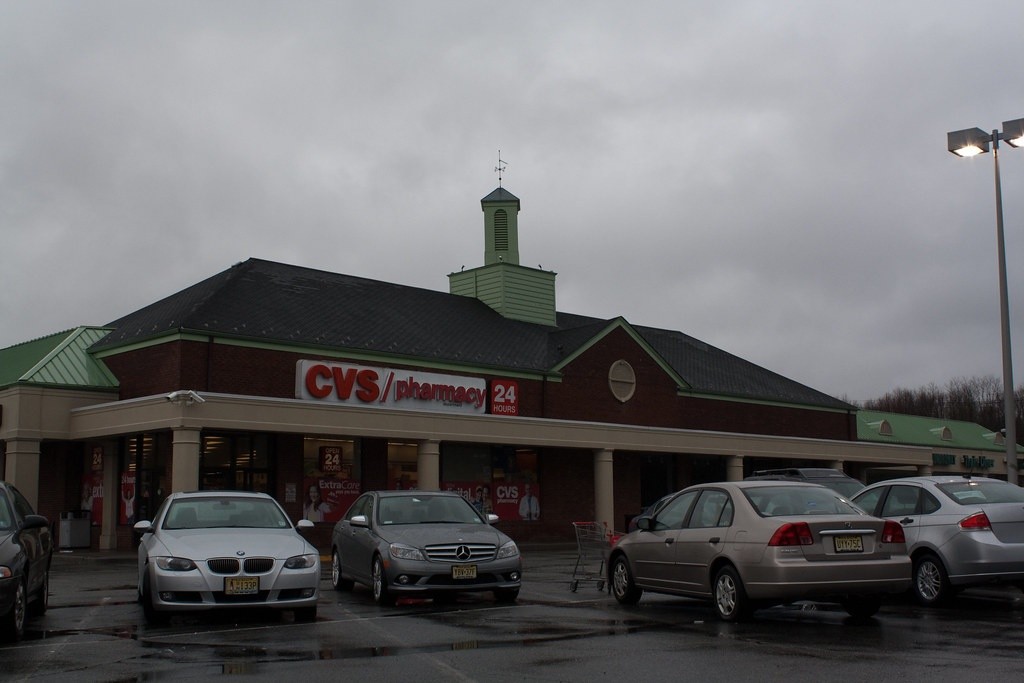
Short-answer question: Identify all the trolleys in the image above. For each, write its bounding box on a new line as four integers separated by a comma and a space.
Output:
568, 520, 627, 593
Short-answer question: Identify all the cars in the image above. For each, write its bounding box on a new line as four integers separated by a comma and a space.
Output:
845, 476, 1024, 607
132, 491, 322, 624
331, 489, 524, 607
0, 480, 53, 638
606, 481, 914, 624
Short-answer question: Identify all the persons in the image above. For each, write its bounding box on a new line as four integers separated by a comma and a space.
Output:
472, 485, 492, 518
80, 481, 92, 510
518, 481, 540, 521
122, 484, 135, 524
303, 484, 329, 522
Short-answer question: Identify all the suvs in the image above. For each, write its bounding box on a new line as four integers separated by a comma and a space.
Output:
741, 466, 879, 514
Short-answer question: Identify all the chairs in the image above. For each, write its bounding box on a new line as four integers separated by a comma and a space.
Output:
177, 508, 274, 527
770, 505, 792, 516
888, 488, 916, 514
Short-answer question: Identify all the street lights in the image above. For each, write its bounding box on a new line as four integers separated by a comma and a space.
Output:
945, 117, 1024, 488
